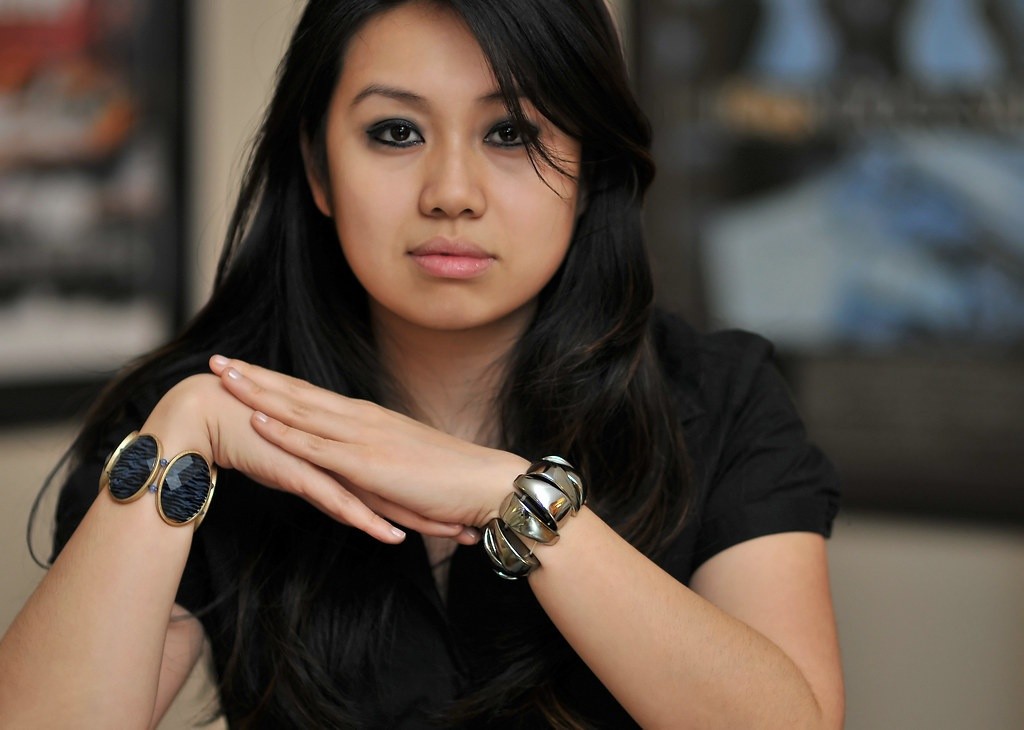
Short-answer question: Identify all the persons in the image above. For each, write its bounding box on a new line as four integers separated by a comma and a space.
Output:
0, 1, 850, 730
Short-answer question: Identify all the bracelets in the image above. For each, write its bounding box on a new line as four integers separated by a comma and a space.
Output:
99, 430, 220, 531
480, 455, 586, 579
